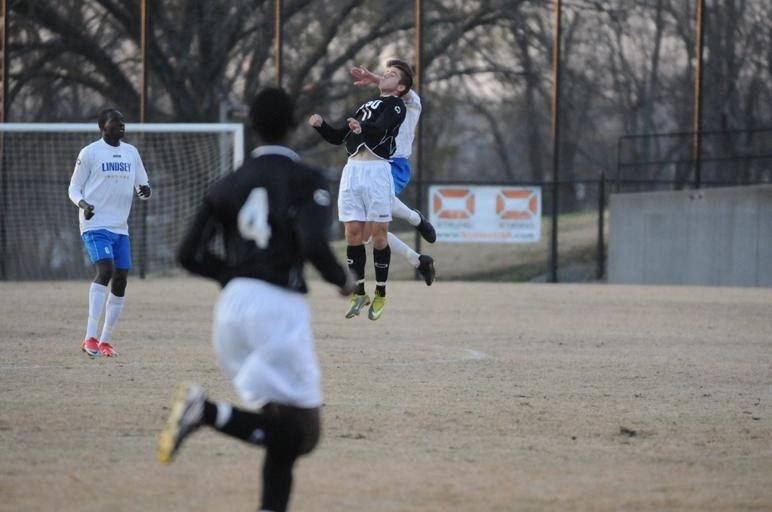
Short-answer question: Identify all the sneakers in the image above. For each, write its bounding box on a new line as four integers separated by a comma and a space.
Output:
417, 254, 435, 287
345, 292, 370, 320
158, 381, 208, 465
368, 296, 386, 321
81, 338, 100, 358
97, 343, 118, 357
413, 208, 436, 244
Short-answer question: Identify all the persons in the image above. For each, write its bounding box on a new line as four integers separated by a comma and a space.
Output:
65, 107, 153, 357
156, 85, 362, 512
349, 60, 438, 289
309, 68, 411, 322
